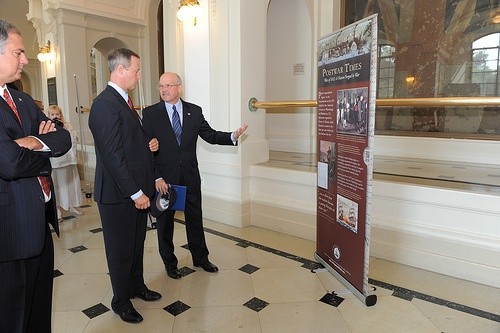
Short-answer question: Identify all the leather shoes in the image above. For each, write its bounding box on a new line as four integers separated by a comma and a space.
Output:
121, 307, 143, 323
193, 262, 218, 272
140, 289, 162, 300
167, 269, 181, 279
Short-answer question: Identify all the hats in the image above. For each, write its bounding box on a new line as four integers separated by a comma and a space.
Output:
150, 186, 176, 218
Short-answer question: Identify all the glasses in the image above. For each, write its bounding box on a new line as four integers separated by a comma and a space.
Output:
156, 83, 179, 91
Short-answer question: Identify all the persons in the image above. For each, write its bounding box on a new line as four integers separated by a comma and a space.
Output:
327, 146, 335, 177
89, 48, 163, 324
141, 72, 248, 279
35, 99, 84, 223
0, 19, 72, 333
339, 96, 367, 126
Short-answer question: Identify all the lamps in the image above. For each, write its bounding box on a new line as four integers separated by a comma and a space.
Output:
177, 0, 201, 26
37, 40, 55, 63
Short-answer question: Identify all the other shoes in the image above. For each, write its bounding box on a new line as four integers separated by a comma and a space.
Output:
70, 207, 84, 215
58, 208, 63, 223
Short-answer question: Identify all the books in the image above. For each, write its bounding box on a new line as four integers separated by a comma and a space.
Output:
155, 185, 186, 211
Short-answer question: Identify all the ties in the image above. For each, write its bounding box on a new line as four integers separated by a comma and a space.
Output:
128, 95, 136, 112
3, 89, 23, 125
172, 105, 183, 144
